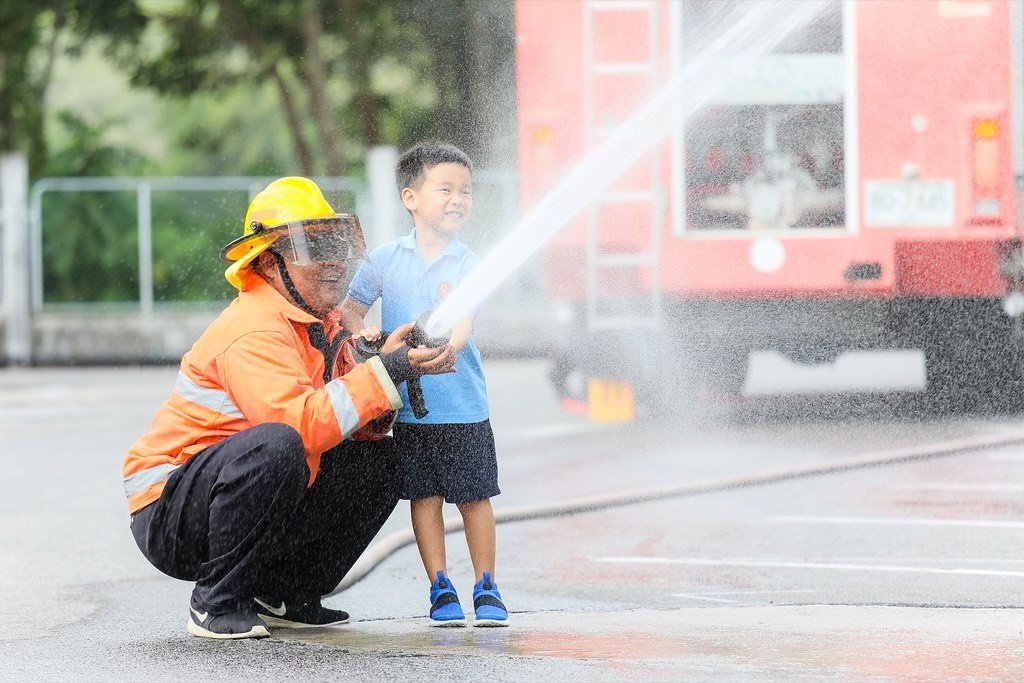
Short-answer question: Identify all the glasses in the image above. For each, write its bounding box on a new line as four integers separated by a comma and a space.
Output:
266, 236, 350, 261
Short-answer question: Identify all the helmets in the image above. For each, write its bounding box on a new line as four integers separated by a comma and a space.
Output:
218, 176, 357, 264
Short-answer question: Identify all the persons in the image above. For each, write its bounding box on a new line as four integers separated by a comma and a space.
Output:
339, 144, 509, 627
122, 177, 457, 638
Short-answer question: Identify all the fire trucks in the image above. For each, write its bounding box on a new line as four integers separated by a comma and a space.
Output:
513, 0, 1024, 419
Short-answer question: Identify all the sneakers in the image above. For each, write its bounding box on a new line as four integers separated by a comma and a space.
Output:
429, 571, 467, 627
186, 596, 271, 638
473, 571, 509, 626
253, 594, 350, 627
